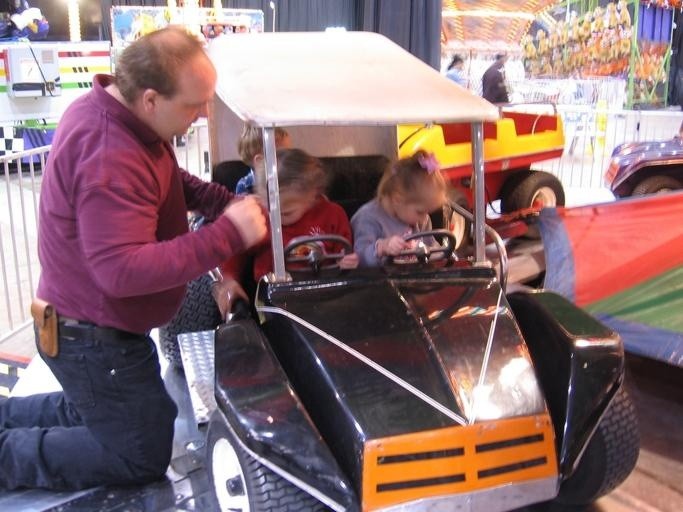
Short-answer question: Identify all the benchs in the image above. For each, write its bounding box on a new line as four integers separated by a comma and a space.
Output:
214, 159, 386, 203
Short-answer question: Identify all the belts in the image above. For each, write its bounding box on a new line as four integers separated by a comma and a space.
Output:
54, 324, 145, 340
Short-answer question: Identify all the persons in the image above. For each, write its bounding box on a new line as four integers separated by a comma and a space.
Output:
209, 148, 360, 323
444, 53, 470, 89
236, 123, 295, 195
0, 23, 269, 495
348, 150, 457, 270
482, 50, 511, 104
